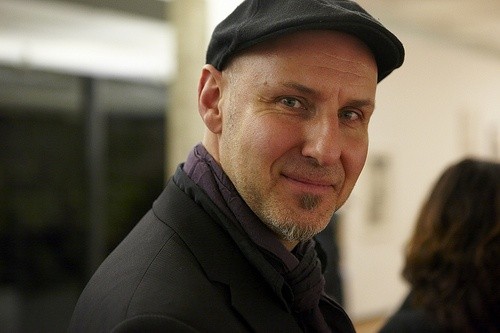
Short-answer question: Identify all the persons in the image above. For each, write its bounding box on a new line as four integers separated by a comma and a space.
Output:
378, 158, 500, 333
65, 0, 405, 333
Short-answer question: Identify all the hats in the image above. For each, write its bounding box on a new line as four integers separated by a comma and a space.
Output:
205, 0, 405, 83
